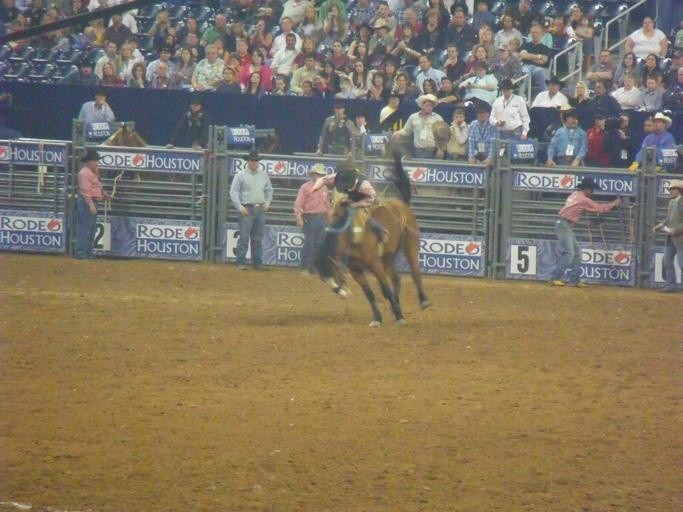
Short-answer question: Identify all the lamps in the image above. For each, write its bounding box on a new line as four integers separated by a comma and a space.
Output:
0, 45, 100, 83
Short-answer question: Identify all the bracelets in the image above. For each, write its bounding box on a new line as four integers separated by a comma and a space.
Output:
538, 54, 541, 59
660, 56, 665, 58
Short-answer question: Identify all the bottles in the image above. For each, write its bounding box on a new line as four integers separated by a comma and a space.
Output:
384, 91, 404, 101
498, 44, 510, 51
336, 170, 357, 193
432, 121, 451, 145
545, 77, 565, 90
74, 59, 97, 66
415, 94, 439, 109
243, 151, 264, 161
307, 163, 328, 175
576, 179, 599, 188
649, 112, 673, 129
373, 18, 392, 32
80, 149, 103, 162
497, 79, 518, 89
664, 179, 683, 189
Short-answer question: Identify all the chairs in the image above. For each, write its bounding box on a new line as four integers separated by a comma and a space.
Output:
552, 279, 589, 287
239, 263, 269, 271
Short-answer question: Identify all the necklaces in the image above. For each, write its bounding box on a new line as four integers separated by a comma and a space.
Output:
624, 70, 632, 74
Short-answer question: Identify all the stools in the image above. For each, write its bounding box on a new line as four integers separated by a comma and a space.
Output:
241, 203, 261, 207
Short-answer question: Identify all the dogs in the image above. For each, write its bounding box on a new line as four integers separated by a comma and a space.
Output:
259, 132, 307, 189
101, 120, 173, 194
311, 146, 435, 329
382, 137, 412, 161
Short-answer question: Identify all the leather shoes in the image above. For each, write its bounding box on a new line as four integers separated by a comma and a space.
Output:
628, 161, 640, 173
656, 166, 662, 173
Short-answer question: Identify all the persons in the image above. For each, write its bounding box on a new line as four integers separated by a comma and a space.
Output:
586, 49, 617, 96
585, 111, 610, 167
489, 79, 530, 140
545, 110, 588, 168
603, 112, 641, 168
549, 179, 621, 287
392, 93, 445, 160
468, 102, 497, 168
639, 53, 663, 90
60, 0, 606, 111
1, 1, 123, 56
630, 111, 681, 167
369, 92, 407, 134
229, 152, 273, 270
314, 96, 359, 159
75, 152, 112, 259
653, 179, 683, 292
163, 95, 213, 149
312, 168, 375, 207
294, 164, 330, 275
78, 87, 116, 133
444, 108, 468, 161
354, 110, 377, 133
1, 91, 21, 131
625, 15, 668, 65
612, 51, 641, 91
663, 17, 681, 146
